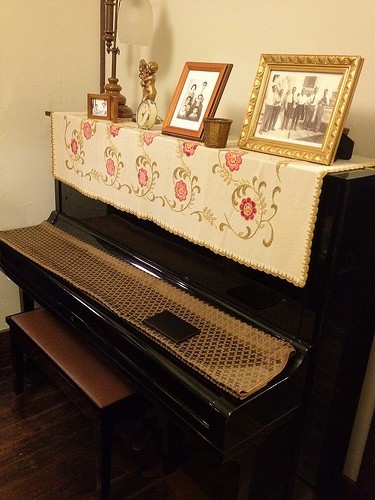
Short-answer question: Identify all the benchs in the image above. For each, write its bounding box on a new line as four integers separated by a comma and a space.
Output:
6, 307, 154, 500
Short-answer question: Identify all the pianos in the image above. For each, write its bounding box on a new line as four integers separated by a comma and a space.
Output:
0, 111, 375, 500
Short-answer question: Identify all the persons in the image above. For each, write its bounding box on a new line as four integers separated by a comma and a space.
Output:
137, 59, 159, 101
178, 79, 208, 124
93, 102, 108, 115
259, 74, 329, 132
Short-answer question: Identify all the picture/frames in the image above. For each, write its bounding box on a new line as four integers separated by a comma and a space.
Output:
161, 62, 233, 142
87, 94, 111, 120
238, 54, 365, 166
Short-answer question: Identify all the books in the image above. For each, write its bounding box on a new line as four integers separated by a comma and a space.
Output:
142, 310, 201, 344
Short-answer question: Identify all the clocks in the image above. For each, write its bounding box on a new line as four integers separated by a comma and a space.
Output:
136, 100, 157, 130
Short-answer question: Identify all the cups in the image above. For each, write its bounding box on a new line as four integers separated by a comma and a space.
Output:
203, 117, 233, 148
